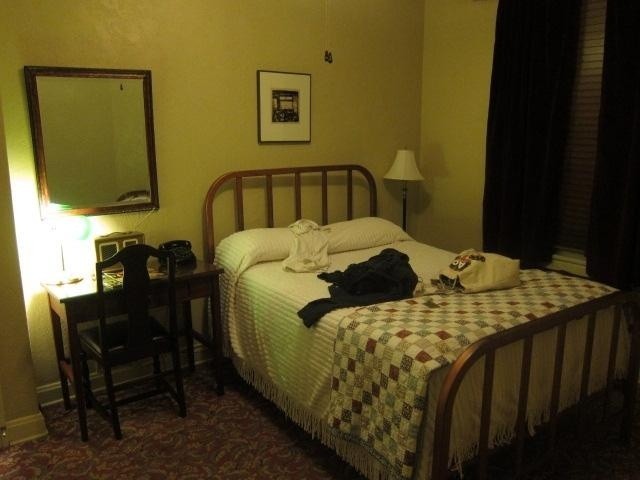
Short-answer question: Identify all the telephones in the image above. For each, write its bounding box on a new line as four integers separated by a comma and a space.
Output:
160, 240, 196, 270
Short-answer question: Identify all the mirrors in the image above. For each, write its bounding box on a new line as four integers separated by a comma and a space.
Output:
23, 64, 159, 221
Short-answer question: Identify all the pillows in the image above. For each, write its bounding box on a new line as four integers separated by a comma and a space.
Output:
208, 216, 414, 352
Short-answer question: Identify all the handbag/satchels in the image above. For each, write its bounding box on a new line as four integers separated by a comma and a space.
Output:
439, 248, 521, 294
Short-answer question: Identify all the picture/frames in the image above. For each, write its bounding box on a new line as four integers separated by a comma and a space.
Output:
256, 68, 312, 143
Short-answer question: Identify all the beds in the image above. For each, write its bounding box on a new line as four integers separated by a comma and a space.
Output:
203, 162, 639, 479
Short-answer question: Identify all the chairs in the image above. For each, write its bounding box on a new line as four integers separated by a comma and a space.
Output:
76, 243, 186, 440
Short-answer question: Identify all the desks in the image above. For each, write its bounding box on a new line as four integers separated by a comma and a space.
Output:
41, 258, 224, 443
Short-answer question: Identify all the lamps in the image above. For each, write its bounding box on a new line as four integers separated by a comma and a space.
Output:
383, 146, 424, 233
